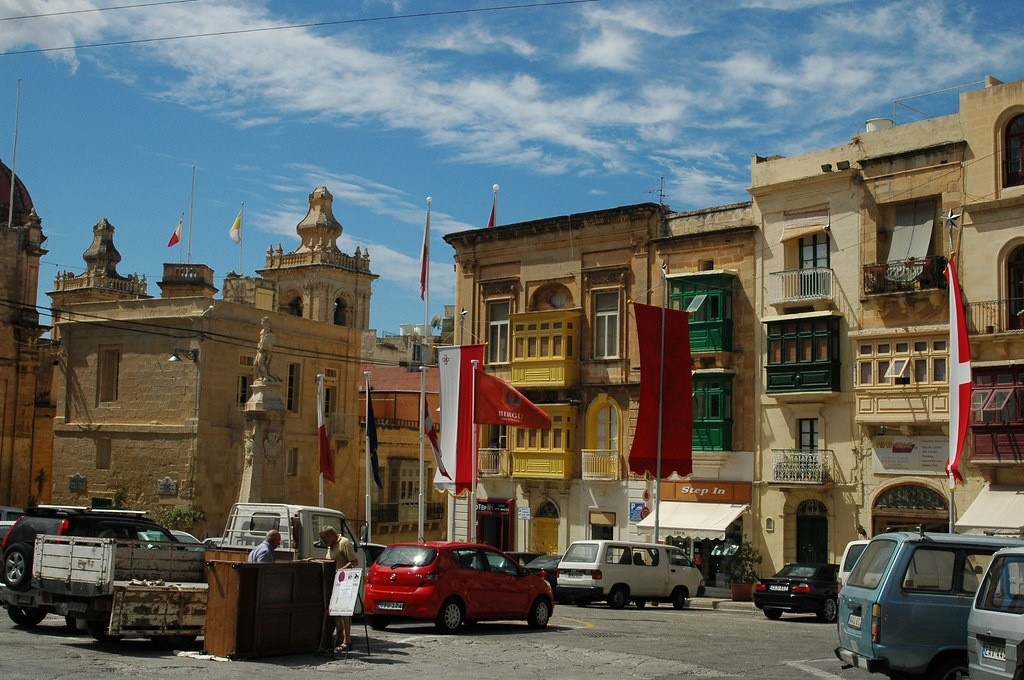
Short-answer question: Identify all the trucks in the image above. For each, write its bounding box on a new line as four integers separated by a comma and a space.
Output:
33, 501, 371, 647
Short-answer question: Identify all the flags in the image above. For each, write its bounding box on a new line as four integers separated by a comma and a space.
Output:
420, 384, 453, 481
487, 198, 495, 227
229, 210, 242, 244
365, 383, 385, 491
419, 211, 427, 301
167, 219, 182, 247
474, 371, 552, 429
316, 382, 336, 484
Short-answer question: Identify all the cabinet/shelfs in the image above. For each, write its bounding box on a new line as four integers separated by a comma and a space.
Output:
198, 558, 333, 658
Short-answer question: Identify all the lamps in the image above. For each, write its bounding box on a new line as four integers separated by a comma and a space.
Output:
821, 161, 849, 172
857, 523, 866, 536
169, 346, 198, 363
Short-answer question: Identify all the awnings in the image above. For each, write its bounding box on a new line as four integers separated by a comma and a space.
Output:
954, 481, 1024, 534
636, 501, 749, 541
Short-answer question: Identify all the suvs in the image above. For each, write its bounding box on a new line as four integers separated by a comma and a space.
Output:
0, 504, 190, 636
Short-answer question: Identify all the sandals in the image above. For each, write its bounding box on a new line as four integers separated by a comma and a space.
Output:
334, 643, 354, 651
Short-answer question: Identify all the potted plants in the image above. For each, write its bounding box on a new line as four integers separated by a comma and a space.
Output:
722, 531, 763, 602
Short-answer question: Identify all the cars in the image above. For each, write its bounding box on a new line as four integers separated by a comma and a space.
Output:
134, 530, 229, 554
0, 506, 26, 544
486, 551, 575, 606
753, 563, 840, 624
364, 541, 555, 635
349, 541, 414, 617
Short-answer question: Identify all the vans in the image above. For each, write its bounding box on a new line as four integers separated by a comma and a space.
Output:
835, 522, 1024, 680
837, 539, 983, 605
555, 539, 705, 610
967, 548, 1024, 680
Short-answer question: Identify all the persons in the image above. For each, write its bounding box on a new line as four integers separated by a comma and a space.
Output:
319, 525, 359, 653
248, 529, 281, 562
